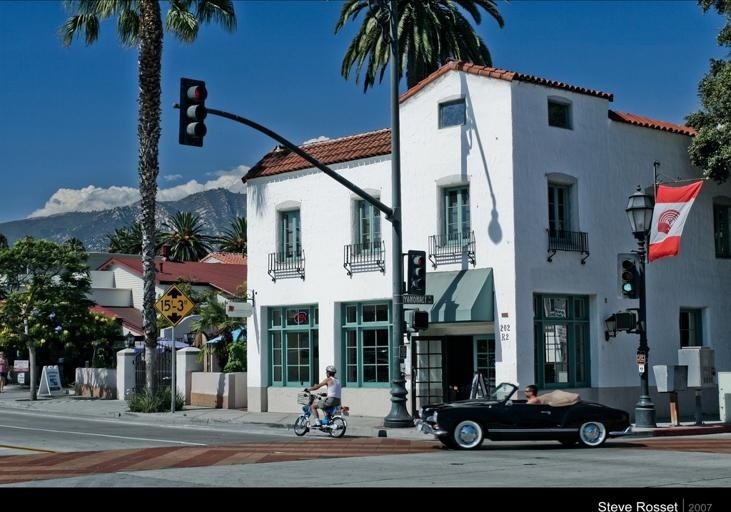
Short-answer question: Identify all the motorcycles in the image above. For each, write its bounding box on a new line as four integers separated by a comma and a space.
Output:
293, 388, 350, 438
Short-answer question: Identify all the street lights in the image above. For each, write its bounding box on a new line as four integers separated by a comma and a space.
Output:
625, 184, 657, 428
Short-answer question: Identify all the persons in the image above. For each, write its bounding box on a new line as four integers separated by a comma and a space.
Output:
0, 351, 10, 395
525, 384, 543, 404
305, 366, 341, 427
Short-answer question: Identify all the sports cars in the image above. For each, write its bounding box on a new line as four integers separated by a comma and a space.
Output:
413, 383, 630, 450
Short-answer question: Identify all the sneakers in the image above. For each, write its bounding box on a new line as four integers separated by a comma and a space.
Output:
311, 422, 322, 426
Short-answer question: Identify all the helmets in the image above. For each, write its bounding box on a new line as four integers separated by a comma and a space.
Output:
326, 366, 337, 373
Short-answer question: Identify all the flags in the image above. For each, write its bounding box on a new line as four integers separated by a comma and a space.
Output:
649, 181, 705, 260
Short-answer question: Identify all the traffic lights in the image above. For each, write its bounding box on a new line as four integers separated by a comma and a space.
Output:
411, 310, 429, 331
616, 252, 641, 300
407, 250, 426, 296
178, 77, 207, 148
614, 311, 637, 332
